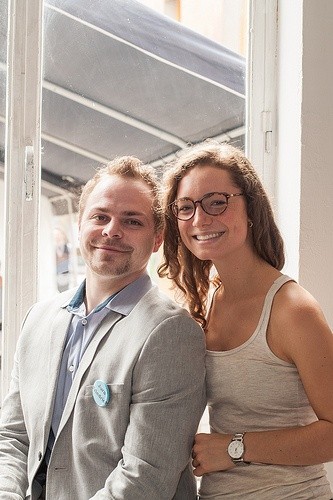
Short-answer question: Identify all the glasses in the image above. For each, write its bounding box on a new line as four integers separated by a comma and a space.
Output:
171, 192, 245, 221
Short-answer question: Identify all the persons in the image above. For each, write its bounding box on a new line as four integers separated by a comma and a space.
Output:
0, 156, 208, 500
156, 143, 333, 500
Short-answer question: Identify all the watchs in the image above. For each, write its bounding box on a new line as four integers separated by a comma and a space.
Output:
226, 432, 251, 466
